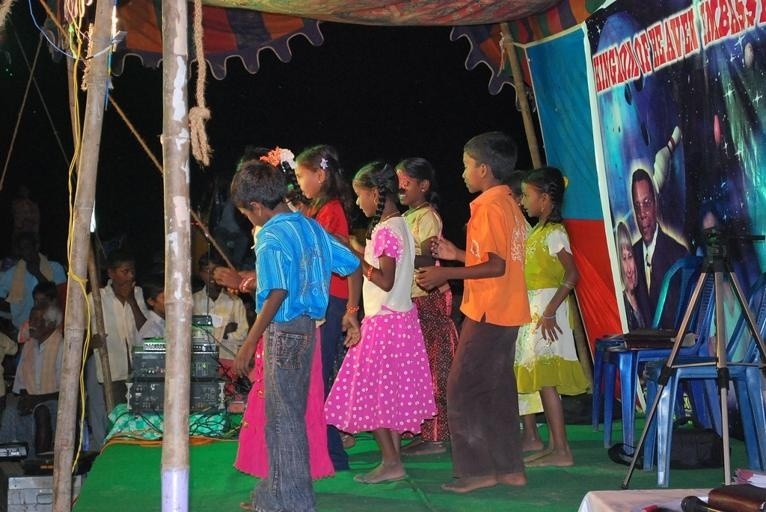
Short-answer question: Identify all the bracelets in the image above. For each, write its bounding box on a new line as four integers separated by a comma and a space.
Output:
542, 313, 557, 320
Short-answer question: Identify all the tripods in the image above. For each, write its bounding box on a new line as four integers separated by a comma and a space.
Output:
621, 259, 766, 486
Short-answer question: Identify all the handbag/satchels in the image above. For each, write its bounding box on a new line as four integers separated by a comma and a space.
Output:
655, 416, 723, 469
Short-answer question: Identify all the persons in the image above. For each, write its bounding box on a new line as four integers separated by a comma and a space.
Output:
628, 167, 691, 323
392, 151, 462, 461
510, 164, 595, 471
414, 127, 534, 494
315, 157, 440, 486
0, 143, 399, 512
511, 392, 568, 453
612, 222, 655, 326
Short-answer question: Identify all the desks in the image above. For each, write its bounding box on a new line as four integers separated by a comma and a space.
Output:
576, 487, 728, 512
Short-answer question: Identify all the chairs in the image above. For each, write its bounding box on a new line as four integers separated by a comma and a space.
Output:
641, 271, 765, 484
595, 254, 723, 458
